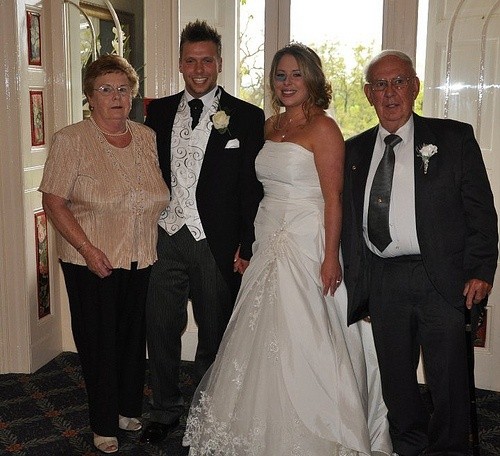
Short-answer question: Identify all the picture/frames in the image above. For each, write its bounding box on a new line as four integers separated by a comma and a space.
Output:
30, 90, 45, 146
34, 213, 52, 319
26, 10, 41, 66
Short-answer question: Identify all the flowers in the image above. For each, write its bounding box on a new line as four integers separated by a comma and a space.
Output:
208, 109, 230, 135
80, 24, 148, 107
415, 141, 438, 176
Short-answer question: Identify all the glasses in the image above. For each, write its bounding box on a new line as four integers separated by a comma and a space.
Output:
93, 84, 132, 96
369, 75, 414, 92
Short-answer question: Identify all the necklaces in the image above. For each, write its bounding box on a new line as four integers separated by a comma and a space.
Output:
282, 111, 304, 139
89, 115, 136, 169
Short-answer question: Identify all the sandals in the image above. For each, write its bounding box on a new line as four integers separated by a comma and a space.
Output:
117, 414, 143, 431
94, 430, 118, 453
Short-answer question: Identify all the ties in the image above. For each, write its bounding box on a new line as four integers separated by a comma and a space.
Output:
367, 134, 402, 253
188, 99, 204, 130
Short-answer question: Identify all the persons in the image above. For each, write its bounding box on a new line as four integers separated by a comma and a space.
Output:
143, 19, 266, 444
36, 54, 170, 454
181, 43, 397, 456
343, 49, 498, 456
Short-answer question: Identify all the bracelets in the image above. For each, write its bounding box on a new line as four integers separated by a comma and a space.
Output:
76, 238, 90, 251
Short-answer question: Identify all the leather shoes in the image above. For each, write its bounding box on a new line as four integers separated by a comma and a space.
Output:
140, 418, 181, 445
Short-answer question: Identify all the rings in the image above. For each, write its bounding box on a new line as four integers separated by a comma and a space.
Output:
336, 280, 341, 283
